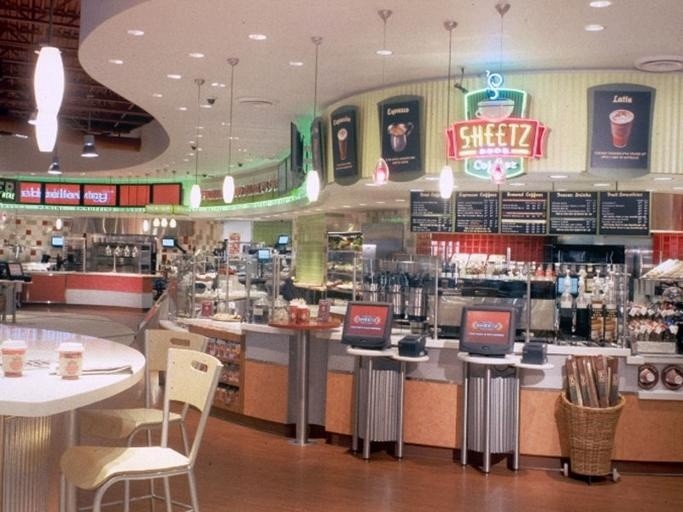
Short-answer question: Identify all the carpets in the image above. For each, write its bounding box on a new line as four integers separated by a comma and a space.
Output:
0, 308, 135, 346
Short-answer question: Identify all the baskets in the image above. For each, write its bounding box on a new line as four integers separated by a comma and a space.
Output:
560, 388, 627, 478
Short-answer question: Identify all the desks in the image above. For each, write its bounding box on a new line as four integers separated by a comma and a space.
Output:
0, 322, 134, 512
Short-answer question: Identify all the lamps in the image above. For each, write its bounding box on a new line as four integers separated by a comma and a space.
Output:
45, 90, 100, 177
371, 4, 393, 187
303, 34, 326, 203
189, 58, 239, 209
438, 1, 511, 204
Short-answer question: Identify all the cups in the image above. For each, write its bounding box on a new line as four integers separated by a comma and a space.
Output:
336, 128, 347, 161
475, 99, 515, 122
57, 343, 83, 382
608, 110, 634, 148
286, 305, 310, 324
386, 121, 414, 153
666, 371, 683, 387
638, 367, 656, 386
0, 339, 27, 377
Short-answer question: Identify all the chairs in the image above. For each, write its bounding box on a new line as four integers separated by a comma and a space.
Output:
58, 327, 224, 512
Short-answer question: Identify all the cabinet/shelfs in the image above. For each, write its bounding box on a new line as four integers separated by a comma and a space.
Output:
185, 323, 243, 415
324, 229, 364, 299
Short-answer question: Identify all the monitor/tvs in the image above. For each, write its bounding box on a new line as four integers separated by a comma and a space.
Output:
460, 306, 516, 358
7, 262, 24, 277
278, 235, 289, 245
51, 236, 63, 247
556, 274, 578, 297
342, 301, 394, 350
163, 238, 174, 248
258, 249, 270, 260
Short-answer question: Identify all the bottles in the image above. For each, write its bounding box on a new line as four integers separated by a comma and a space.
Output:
429, 240, 619, 344
